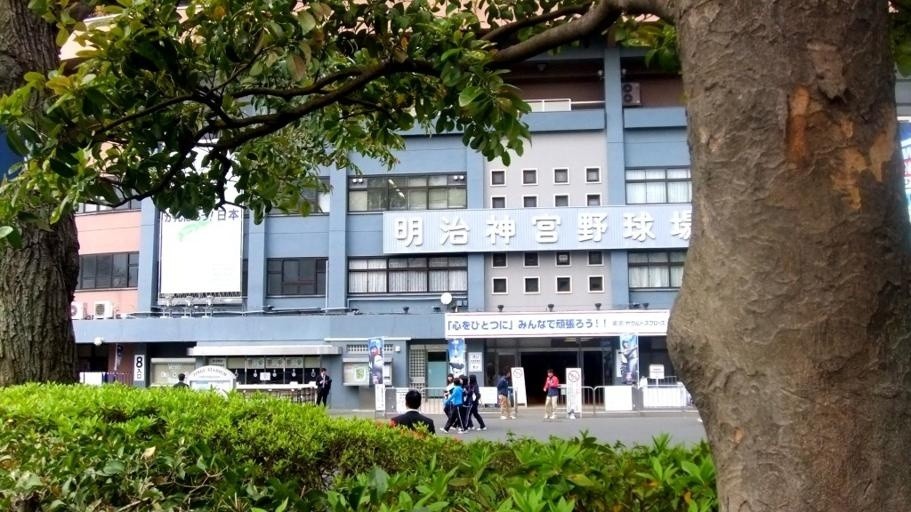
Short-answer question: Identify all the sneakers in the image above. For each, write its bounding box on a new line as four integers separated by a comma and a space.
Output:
510, 416, 516, 420
501, 416, 507, 420
440, 426, 486, 434
544, 415, 549, 418
550, 415, 558, 419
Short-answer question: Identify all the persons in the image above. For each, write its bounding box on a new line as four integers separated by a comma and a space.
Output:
174, 373, 189, 389
314, 367, 333, 407
368, 340, 384, 385
497, 370, 516, 420
439, 373, 486, 434
543, 368, 560, 418
622, 340, 637, 384
391, 389, 435, 434
